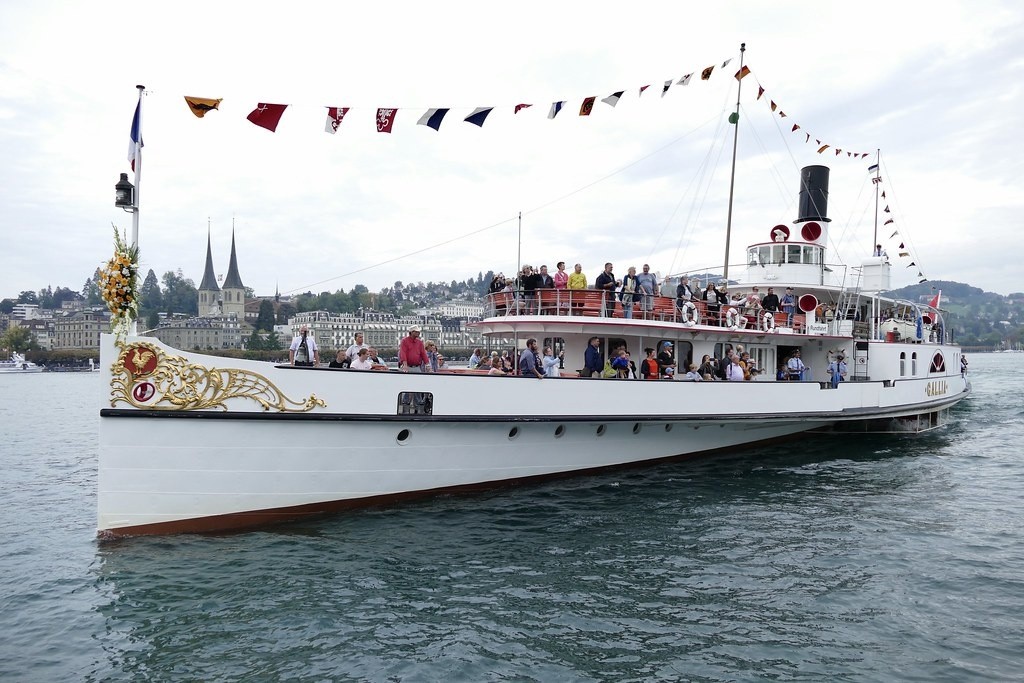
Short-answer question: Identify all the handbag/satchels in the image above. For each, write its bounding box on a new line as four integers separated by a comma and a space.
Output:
580, 368, 592, 376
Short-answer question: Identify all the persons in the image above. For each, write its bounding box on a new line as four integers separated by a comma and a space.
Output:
581, 337, 677, 380
487, 261, 795, 328
778, 348, 810, 381
827, 355, 848, 381
875, 311, 942, 341
328, 332, 389, 371
469, 338, 565, 378
289, 326, 319, 368
961, 355, 968, 372
398, 325, 448, 372
687, 345, 766, 381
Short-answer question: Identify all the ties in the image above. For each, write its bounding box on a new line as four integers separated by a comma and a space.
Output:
797, 359, 799, 373
837, 363, 840, 372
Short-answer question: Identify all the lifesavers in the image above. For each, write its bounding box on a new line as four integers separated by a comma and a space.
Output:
762, 312, 775, 334
726, 307, 741, 331
682, 301, 699, 328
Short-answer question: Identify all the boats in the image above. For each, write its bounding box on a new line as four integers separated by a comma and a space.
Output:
992, 348, 1024, 353
0, 354, 45, 372
97, 229, 973, 542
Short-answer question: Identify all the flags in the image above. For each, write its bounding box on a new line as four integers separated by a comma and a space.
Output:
325, 106, 351, 135
868, 164, 936, 290
928, 293, 938, 321
127, 98, 144, 171
376, 108, 398, 133
185, 96, 223, 118
415, 58, 732, 132
247, 103, 289, 133
734, 65, 869, 159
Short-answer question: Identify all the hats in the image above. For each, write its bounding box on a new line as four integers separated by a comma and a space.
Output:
666, 368, 675, 373
787, 287, 794, 290
664, 342, 674, 347
409, 325, 421, 332
837, 356, 844, 360
792, 349, 799, 353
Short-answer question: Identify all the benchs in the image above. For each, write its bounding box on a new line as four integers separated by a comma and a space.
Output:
435, 369, 490, 374
486, 291, 806, 333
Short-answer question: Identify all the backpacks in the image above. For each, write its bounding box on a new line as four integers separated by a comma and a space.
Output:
595, 274, 603, 288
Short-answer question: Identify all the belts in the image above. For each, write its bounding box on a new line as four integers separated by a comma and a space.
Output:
410, 365, 419, 367
